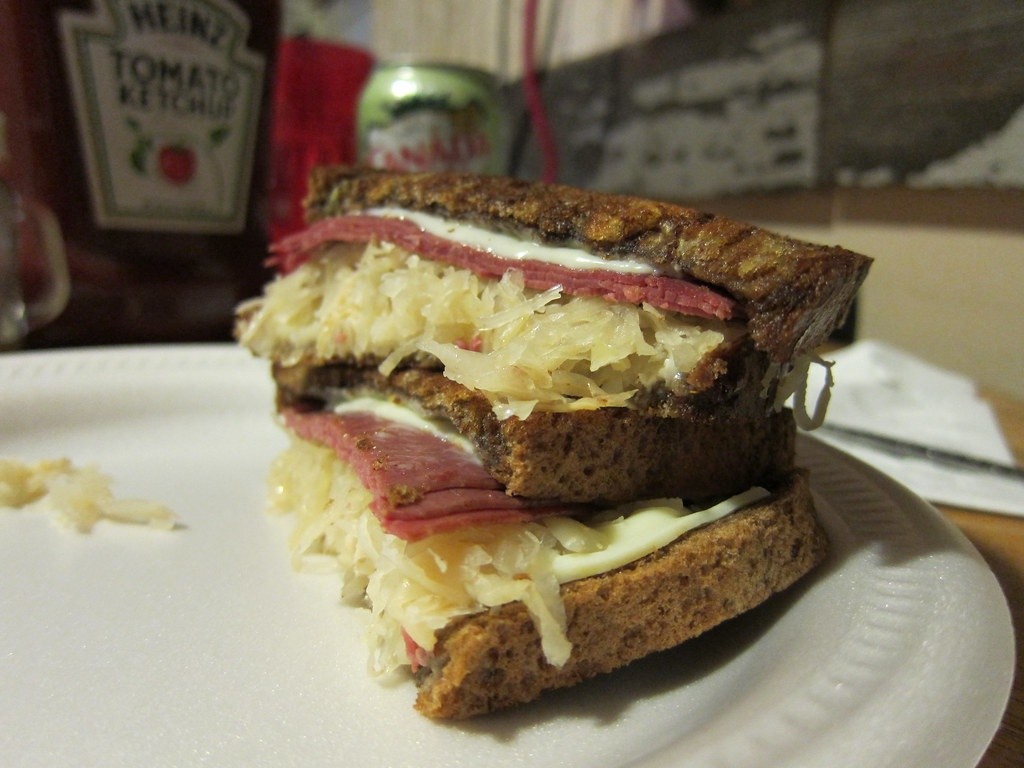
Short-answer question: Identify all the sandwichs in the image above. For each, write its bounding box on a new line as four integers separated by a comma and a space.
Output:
231, 162, 877, 723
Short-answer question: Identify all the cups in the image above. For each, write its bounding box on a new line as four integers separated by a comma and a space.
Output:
271, 0, 380, 273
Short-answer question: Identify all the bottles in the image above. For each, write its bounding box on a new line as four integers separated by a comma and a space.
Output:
0, 0, 276, 347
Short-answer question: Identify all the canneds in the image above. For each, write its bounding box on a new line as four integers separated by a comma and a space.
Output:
351, 61, 516, 181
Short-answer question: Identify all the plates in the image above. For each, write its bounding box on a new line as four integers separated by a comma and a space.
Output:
0, 345, 1016, 768
